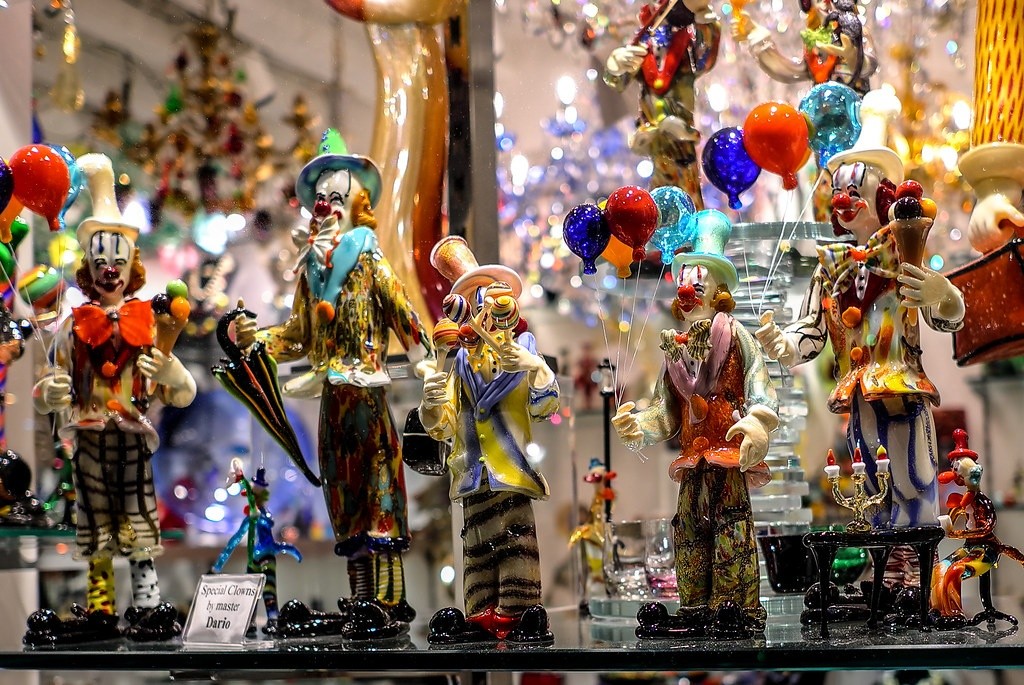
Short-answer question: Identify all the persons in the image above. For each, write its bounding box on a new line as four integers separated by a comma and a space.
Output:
612, 252, 781, 615
33, 230, 198, 615
236, 165, 435, 622
731, 0, 880, 220
931, 429, 1002, 619
757, 149, 964, 534
420, 275, 561, 628
602, 0, 721, 212
959, 1, 1024, 255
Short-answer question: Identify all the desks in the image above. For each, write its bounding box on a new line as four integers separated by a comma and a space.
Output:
0, 611, 1024, 669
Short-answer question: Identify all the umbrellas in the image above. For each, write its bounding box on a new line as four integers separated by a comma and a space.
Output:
210, 308, 324, 487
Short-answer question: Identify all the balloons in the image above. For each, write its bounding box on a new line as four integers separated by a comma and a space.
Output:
561, 186, 696, 275
704, 82, 862, 212
0, 145, 87, 313
